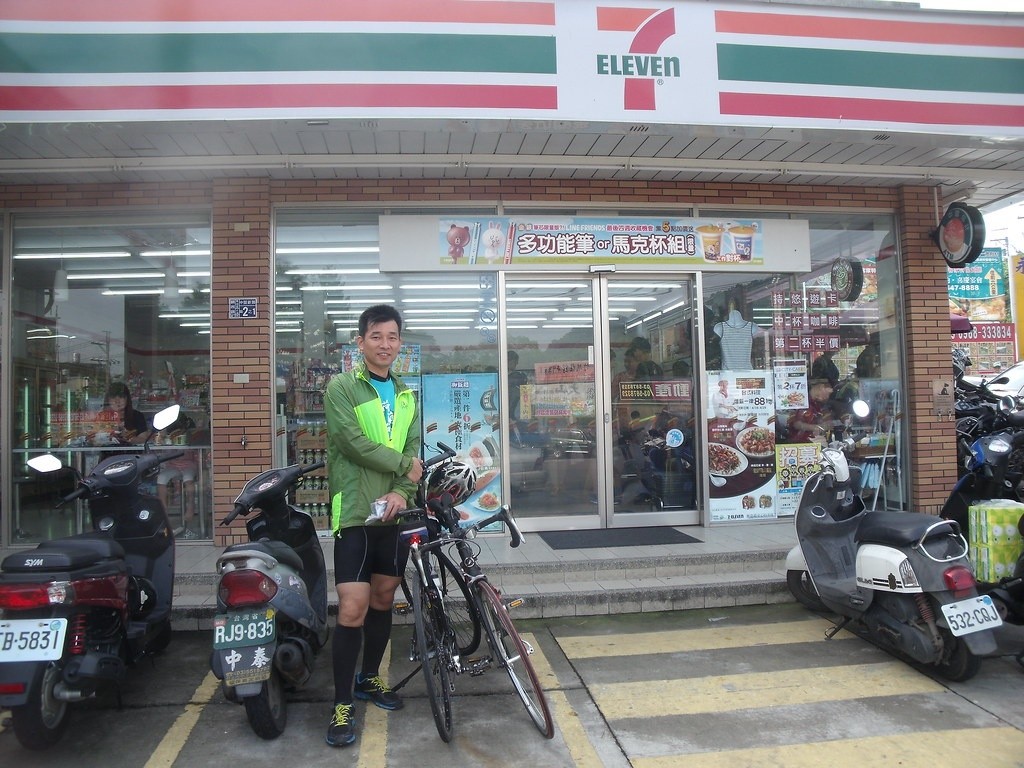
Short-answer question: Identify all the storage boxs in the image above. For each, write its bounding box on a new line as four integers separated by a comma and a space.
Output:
843, 444, 894, 457
299, 464, 328, 477
295, 437, 327, 450
295, 489, 330, 504
312, 516, 329, 529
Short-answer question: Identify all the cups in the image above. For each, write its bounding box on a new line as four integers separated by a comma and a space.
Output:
728, 226, 755, 263
696, 226, 725, 264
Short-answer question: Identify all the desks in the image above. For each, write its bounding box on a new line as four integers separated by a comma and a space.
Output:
14, 444, 211, 541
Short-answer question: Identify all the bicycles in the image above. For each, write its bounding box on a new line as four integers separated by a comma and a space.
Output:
388, 442, 555, 743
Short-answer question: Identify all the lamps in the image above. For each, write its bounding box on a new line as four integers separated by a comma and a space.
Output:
53, 259, 68, 300
164, 256, 179, 297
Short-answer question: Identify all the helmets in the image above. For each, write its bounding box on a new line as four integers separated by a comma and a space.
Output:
417, 458, 476, 508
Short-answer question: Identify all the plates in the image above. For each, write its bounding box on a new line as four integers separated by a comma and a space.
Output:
708, 442, 749, 476
470, 491, 501, 512
736, 427, 775, 458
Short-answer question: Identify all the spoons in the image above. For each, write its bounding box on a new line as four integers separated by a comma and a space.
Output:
710, 474, 727, 488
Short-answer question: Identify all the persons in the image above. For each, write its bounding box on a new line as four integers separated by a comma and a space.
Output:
544, 337, 689, 508
992, 361, 1002, 373
95, 382, 149, 444
508, 351, 527, 498
965, 366, 972, 375
712, 372, 738, 418
323, 304, 424, 746
156, 411, 197, 521
856, 331, 880, 377
789, 378, 833, 441
813, 351, 839, 385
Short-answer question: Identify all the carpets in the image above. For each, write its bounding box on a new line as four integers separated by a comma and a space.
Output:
538, 526, 706, 550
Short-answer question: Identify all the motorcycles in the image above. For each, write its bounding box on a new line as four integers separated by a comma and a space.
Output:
644, 409, 695, 510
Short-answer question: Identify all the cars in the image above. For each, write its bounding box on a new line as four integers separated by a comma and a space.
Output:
983, 361, 1024, 396
542, 428, 597, 458
510, 441, 546, 505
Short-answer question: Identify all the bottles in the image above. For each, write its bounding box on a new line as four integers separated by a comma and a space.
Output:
296, 422, 329, 517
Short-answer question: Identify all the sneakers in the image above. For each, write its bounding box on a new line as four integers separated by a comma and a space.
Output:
353, 672, 403, 711
326, 702, 356, 747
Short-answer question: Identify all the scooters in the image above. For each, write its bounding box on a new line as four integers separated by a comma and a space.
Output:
211, 461, 329, 736
0, 405, 185, 749
940, 349, 1024, 626
786, 400, 1003, 682
